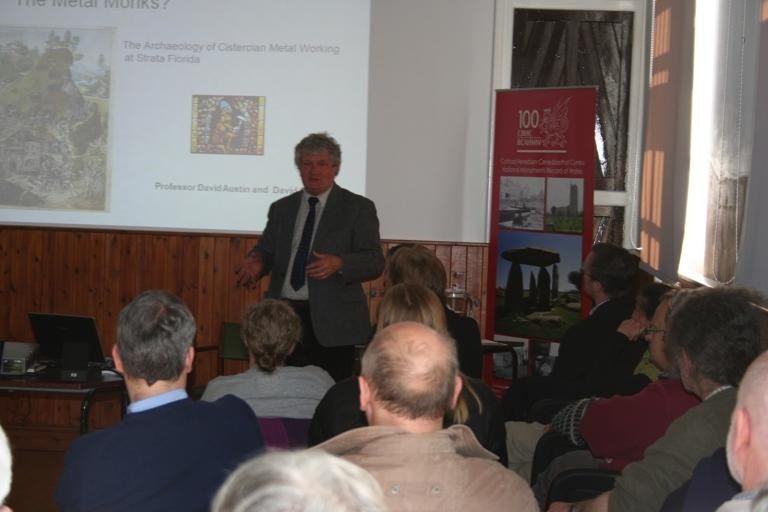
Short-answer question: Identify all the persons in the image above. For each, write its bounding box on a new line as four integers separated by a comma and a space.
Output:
505, 241, 766, 511
231, 132, 379, 379
55, 287, 264, 512
383, 244, 485, 386
306, 286, 508, 455
301, 324, 527, 512
206, 448, 388, 512
198, 295, 337, 424
0, 420, 20, 512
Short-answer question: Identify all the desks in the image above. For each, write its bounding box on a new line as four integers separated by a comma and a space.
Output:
0, 375, 129, 437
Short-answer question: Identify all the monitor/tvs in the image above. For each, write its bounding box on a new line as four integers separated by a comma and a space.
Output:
28, 312, 105, 384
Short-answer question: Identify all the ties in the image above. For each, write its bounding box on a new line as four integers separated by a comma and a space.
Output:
290, 197, 318, 291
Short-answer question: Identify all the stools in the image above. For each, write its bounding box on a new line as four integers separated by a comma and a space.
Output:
481, 338, 518, 388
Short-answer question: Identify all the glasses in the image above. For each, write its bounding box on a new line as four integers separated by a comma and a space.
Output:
577, 267, 593, 280
642, 328, 665, 341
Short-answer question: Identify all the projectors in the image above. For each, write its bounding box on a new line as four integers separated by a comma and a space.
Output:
0, 339, 39, 376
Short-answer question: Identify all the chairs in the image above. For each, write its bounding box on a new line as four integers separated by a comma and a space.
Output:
530, 395, 571, 425
186, 322, 250, 401
530, 431, 587, 488
546, 467, 621, 508
